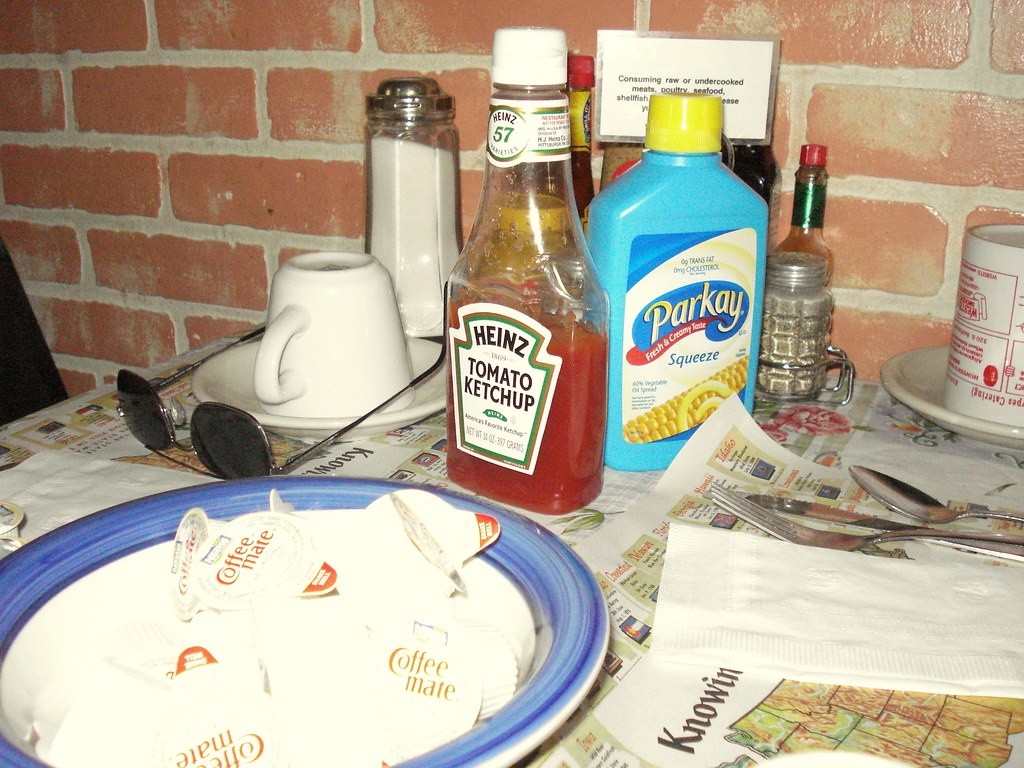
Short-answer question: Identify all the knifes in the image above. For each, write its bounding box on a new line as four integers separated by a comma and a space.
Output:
746, 494, 1024, 563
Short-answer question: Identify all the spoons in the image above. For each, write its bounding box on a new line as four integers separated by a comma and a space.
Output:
848, 464, 1024, 525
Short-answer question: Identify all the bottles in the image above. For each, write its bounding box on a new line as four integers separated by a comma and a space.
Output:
366, 76, 466, 338
771, 145, 833, 286
563, 56, 596, 241
759, 252, 832, 396
445, 27, 609, 515
586, 94, 770, 470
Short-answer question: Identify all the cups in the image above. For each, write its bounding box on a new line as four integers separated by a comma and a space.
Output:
942, 222, 1024, 429
255, 253, 413, 417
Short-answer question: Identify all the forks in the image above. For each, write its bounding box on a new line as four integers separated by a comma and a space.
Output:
711, 481, 1024, 552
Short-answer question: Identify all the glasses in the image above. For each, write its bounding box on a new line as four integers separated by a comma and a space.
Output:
117, 324, 447, 479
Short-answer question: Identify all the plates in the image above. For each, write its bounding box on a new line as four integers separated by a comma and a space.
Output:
879, 345, 1024, 450
1, 475, 610, 768
191, 335, 446, 439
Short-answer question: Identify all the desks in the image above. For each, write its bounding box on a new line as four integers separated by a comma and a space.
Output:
0, 320, 1024, 768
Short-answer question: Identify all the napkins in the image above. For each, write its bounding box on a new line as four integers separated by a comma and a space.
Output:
649, 520, 1024, 702
